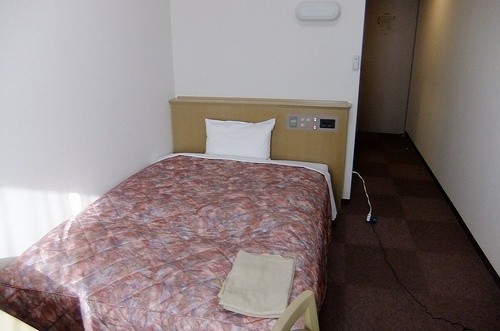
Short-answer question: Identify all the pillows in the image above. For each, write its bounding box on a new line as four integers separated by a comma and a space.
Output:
202, 117, 276, 160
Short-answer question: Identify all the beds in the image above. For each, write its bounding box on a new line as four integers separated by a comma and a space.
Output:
0, 153, 338, 331
